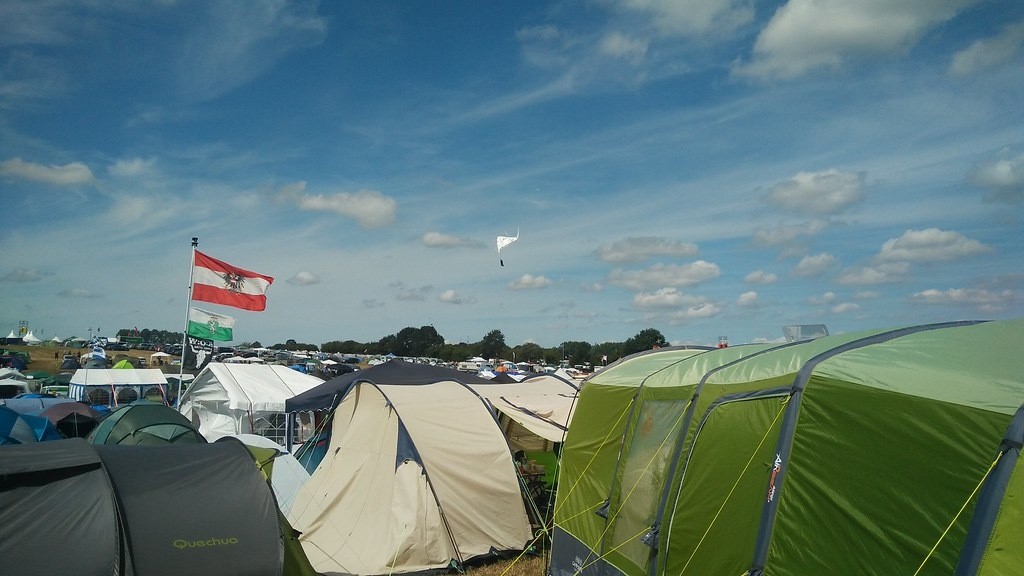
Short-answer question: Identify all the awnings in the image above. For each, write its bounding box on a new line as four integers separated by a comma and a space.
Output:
151, 351, 170, 356
223, 356, 264, 361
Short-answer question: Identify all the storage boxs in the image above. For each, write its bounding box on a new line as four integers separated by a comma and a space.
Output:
534, 465, 546, 473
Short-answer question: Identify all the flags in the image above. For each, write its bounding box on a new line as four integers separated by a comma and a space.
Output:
190, 249, 274, 311
183, 336, 214, 370
185, 307, 235, 341
602, 355, 607, 361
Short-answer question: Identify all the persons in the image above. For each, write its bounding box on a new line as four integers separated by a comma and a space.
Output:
54, 351, 60, 361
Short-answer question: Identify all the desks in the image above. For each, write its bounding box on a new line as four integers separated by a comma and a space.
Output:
523, 472, 546, 493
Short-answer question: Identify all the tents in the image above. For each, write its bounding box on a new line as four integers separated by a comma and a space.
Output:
284, 358, 582, 576
23, 330, 41, 342
178, 362, 327, 443
545, 318, 1024, 576
0, 436, 285, 576
6, 330, 17, 338
0, 347, 208, 446
52, 336, 62, 342
235, 434, 322, 576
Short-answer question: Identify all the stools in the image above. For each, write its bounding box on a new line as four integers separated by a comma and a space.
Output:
528, 481, 547, 497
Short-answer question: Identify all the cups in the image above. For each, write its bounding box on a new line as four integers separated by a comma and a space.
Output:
718, 336, 728, 348
